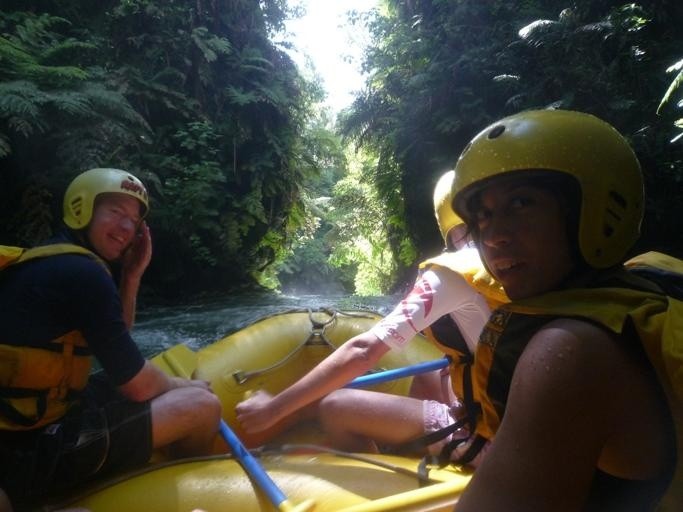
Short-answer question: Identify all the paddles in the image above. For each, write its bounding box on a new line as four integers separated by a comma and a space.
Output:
148, 344, 315, 512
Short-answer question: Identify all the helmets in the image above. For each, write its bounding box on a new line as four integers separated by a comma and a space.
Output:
62, 167, 150, 230
449, 107, 647, 269
431, 168, 466, 249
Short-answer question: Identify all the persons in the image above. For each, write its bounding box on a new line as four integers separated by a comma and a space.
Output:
233, 165, 513, 471
449, 106, 682, 511
1, 169, 223, 511
